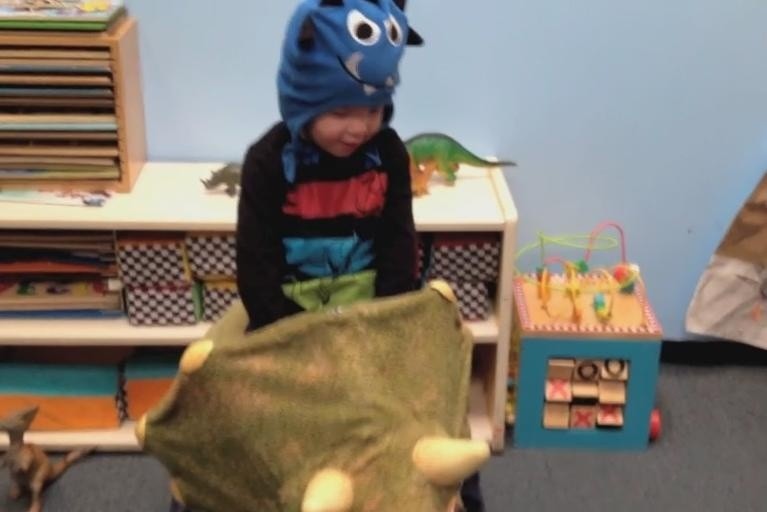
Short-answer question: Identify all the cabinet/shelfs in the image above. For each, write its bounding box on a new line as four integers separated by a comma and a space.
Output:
2, 154, 520, 453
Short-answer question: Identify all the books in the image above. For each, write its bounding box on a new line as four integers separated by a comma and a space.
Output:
0, 1, 123, 179
0, 229, 124, 317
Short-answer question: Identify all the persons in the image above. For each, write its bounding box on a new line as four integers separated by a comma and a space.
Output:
231, 1, 418, 333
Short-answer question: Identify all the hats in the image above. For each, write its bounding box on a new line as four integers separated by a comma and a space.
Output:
272, 0, 426, 184
